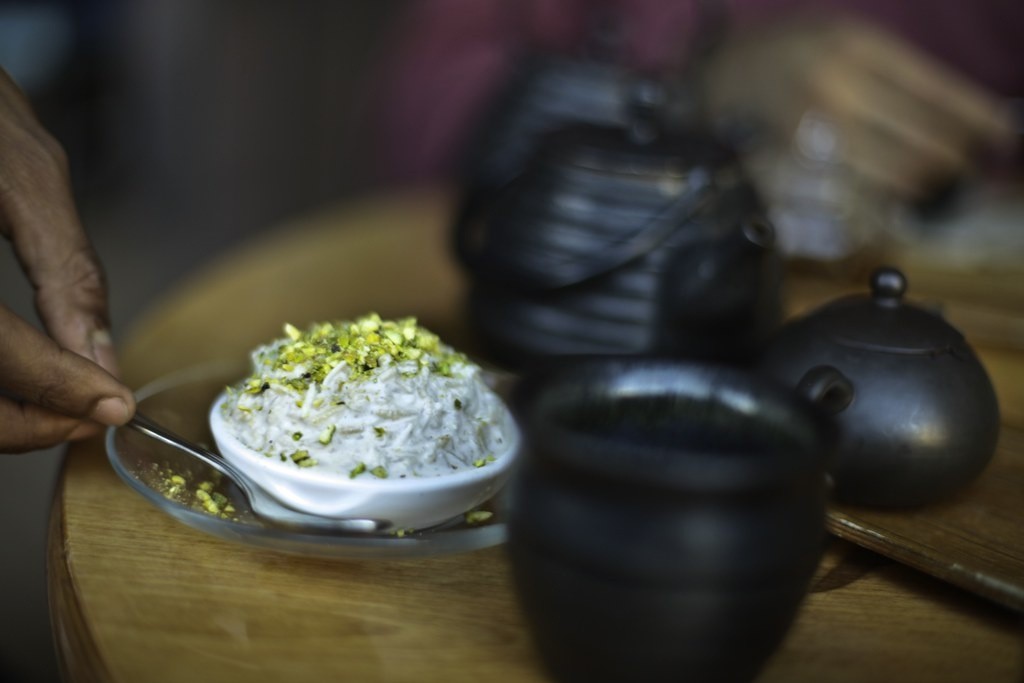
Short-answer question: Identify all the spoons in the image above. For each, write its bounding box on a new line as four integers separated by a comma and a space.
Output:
124, 408, 392, 531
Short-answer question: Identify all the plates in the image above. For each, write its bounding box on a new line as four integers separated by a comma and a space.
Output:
208, 392, 517, 533
104, 367, 518, 555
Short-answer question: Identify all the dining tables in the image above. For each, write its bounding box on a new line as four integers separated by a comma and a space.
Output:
42, 187, 1023, 682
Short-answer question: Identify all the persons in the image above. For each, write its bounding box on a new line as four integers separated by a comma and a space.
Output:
382, 0, 1024, 224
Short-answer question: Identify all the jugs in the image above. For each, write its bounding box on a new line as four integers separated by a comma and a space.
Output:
446, 17, 683, 283
753, 267, 1000, 514
460, 66, 785, 376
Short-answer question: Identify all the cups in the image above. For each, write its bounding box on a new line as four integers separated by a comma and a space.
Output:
506, 357, 855, 683
